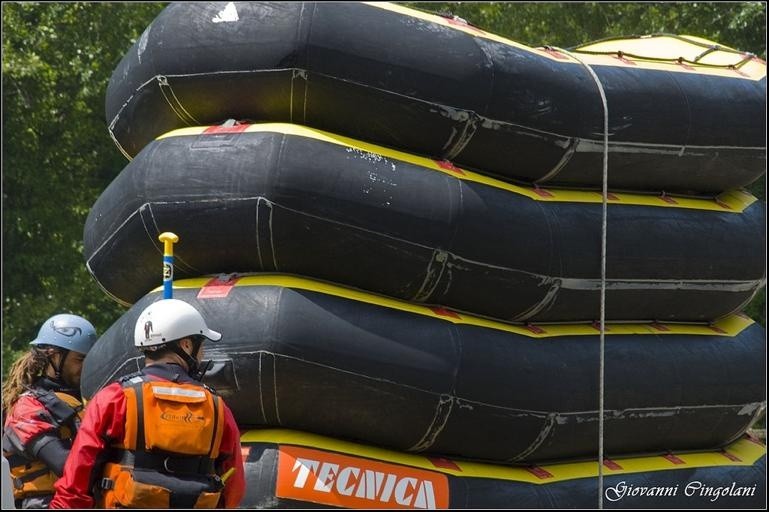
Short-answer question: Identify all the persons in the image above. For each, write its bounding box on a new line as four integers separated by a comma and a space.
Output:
50, 299, 245, 509
1, 314, 98, 509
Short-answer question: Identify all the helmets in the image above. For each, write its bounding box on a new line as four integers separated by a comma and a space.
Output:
29, 314, 97, 355
134, 299, 222, 346
